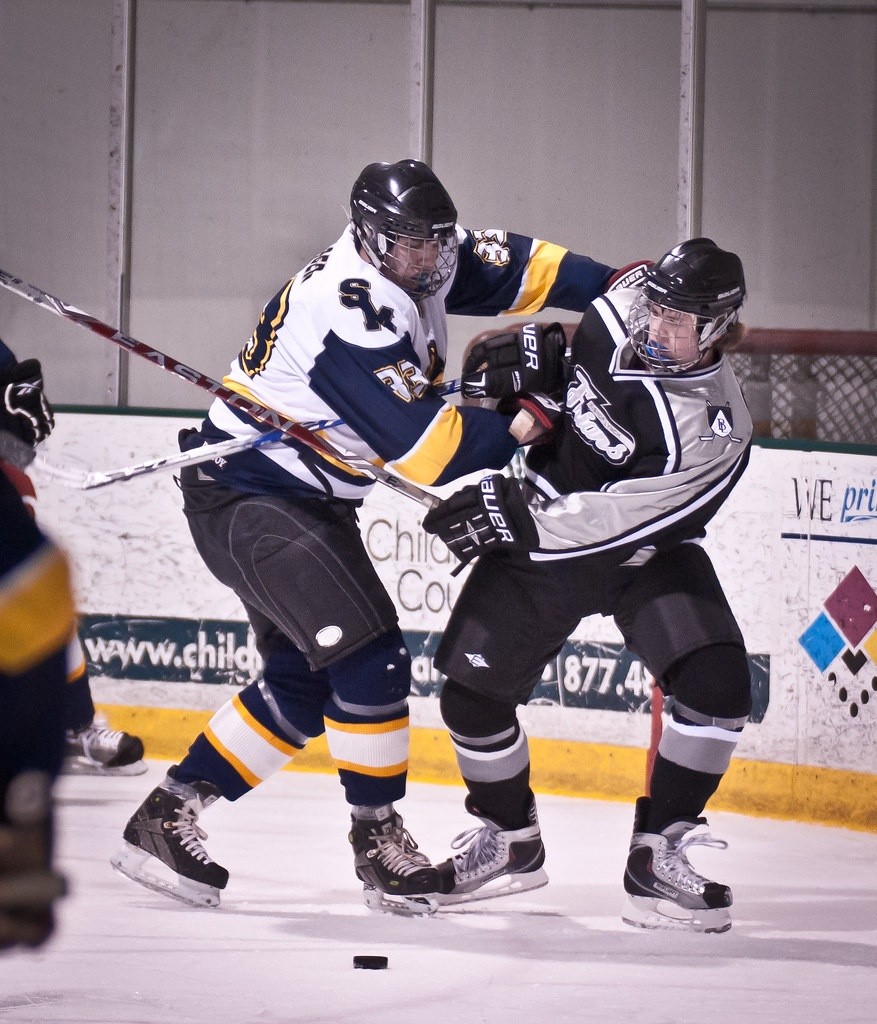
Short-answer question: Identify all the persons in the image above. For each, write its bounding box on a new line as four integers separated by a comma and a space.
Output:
0, 340, 147, 947
421, 224, 752, 934
111, 160, 566, 922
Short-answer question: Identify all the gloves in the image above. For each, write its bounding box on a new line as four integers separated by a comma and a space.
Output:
422, 474, 541, 563
497, 392, 569, 446
461, 322, 568, 399
607, 259, 656, 296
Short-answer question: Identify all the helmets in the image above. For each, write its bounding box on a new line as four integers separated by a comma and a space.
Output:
628, 238, 745, 375
350, 160, 459, 300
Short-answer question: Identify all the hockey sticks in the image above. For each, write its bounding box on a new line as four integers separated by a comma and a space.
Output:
0, 270, 466, 514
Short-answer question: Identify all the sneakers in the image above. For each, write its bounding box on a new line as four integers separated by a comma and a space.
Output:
60, 721, 149, 775
432, 793, 550, 905
348, 811, 438, 917
621, 796, 734, 935
111, 777, 228, 909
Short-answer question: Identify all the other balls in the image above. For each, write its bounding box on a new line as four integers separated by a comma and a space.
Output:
351, 954, 389, 970
